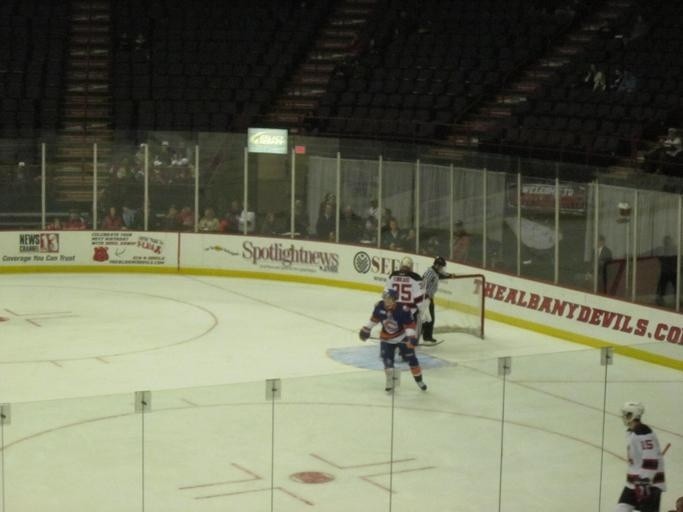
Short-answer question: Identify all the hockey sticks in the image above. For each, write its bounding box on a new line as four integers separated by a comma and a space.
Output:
369, 336, 445, 347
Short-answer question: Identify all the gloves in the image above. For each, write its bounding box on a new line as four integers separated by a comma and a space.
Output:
405, 334, 418, 349
359, 325, 371, 341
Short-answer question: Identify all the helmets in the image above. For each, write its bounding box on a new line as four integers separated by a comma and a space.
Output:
400, 255, 413, 271
381, 286, 399, 300
434, 255, 446, 267
617, 401, 644, 423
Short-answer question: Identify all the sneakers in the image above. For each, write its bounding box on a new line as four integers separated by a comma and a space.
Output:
385, 375, 393, 391
417, 379, 426, 391
423, 336, 436, 342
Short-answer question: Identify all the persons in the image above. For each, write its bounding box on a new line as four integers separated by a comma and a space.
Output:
97, 138, 260, 233
260, 194, 416, 251
643, 129, 680, 177
607, 401, 668, 512
425, 219, 471, 263
386, 255, 433, 365
581, 235, 612, 289
585, 13, 648, 97
653, 236, 677, 307
359, 290, 433, 394
417, 255, 457, 344
46, 213, 87, 231
12, 162, 32, 194
